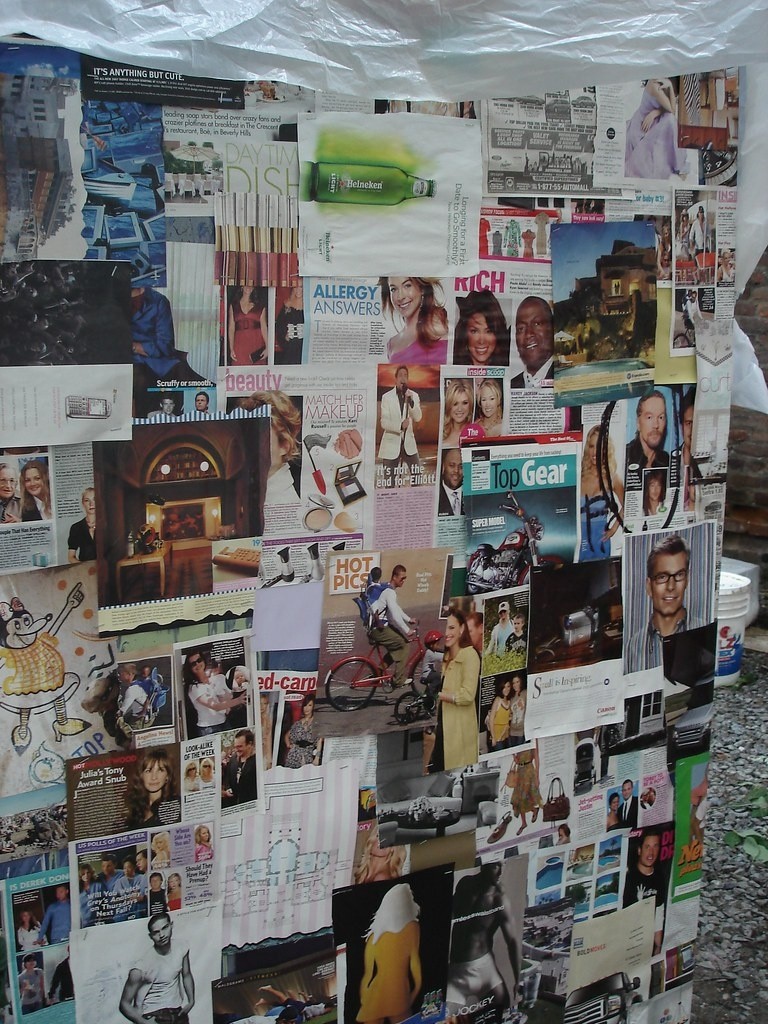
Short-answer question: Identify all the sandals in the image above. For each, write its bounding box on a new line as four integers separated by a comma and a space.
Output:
516, 825, 528, 836
532, 807, 539, 823
485, 811, 513, 844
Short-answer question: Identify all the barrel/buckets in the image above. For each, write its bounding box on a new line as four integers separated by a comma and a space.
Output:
714, 573, 751, 690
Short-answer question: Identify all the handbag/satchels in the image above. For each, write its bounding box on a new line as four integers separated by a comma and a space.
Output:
484, 696, 501, 731
500, 757, 518, 794
543, 777, 571, 822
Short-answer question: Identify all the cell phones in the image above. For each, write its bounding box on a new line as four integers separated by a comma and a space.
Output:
64, 395, 112, 420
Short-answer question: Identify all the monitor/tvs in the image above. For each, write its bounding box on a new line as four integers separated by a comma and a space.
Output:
80, 146, 96, 173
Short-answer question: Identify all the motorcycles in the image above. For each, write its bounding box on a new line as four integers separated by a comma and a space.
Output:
462, 493, 570, 595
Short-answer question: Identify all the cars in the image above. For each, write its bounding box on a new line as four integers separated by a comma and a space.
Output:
560, 971, 644, 1024
667, 685, 713, 755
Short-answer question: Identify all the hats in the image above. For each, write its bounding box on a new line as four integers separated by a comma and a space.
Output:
681, 209, 689, 214
698, 206, 704, 213
22, 953, 37, 962
498, 602, 509, 613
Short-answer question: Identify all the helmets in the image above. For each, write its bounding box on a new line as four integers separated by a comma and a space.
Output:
424, 630, 444, 648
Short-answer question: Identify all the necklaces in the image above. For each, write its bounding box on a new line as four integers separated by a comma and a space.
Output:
89, 526, 96, 528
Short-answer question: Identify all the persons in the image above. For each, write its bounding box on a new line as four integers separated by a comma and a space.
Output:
227, 284, 304, 364
511, 296, 554, 389
240, 390, 302, 501
682, 290, 704, 345
116, 651, 322, 830
131, 286, 216, 419
453, 290, 510, 365
647, 215, 670, 279
675, 206, 711, 284
378, 365, 503, 515
578, 387, 696, 562
0, 805, 214, 1024
717, 248, 735, 282
462, 101, 477, 120
625, 75, 698, 183
253, 536, 710, 1024
378, 277, 449, 363
0, 446, 97, 563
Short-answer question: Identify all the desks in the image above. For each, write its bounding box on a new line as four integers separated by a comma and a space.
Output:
115, 541, 173, 603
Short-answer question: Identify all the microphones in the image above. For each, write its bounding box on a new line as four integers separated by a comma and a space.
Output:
403, 383, 414, 405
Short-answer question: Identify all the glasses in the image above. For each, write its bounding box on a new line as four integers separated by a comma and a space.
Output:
499, 610, 506, 615
190, 657, 204, 668
203, 764, 210, 768
401, 576, 407, 580
187, 767, 196, 772
650, 568, 686, 584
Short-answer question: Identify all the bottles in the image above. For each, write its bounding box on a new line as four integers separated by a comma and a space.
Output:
127, 531, 135, 559
313, 161, 436, 207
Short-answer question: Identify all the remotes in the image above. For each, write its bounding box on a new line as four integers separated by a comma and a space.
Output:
215, 547, 263, 572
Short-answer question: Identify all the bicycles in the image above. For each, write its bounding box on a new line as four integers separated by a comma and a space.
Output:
396, 679, 446, 727
325, 622, 433, 712
